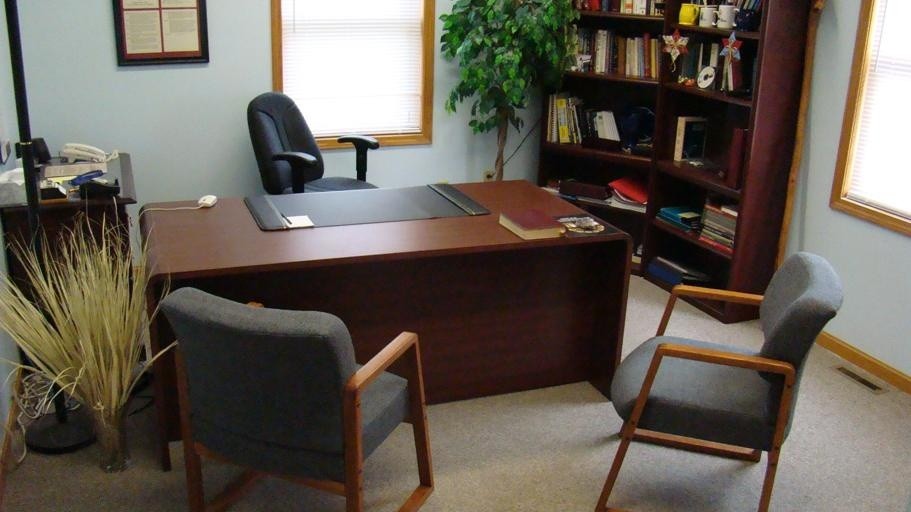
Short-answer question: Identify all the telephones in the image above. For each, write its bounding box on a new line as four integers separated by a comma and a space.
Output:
58, 142, 119, 164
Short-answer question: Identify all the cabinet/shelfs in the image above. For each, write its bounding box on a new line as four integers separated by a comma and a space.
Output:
536, 1, 825, 324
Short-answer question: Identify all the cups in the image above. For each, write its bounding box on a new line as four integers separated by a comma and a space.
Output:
699, 7, 722, 29
679, 3, 700, 26
718, 6, 741, 30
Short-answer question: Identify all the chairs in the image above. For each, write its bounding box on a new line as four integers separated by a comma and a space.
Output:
161, 285, 434, 512
597, 250, 845, 512
247, 91, 382, 195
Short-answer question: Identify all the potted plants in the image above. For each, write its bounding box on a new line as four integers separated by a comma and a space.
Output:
440, 2, 578, 179
1, 198, 175, 472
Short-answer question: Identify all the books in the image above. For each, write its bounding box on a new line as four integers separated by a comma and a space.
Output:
691, 0, 763, 28
575, 29, 660, 81
571, 1, 665, 18
547, 92, 619, 144
655, 203, 738, 253
674, 118, 709, 162
727, 130, 744, 188
678, 41, 745, 92
499, 210, 566, 240
541, 177, 647, 214
658, 256, 708, 287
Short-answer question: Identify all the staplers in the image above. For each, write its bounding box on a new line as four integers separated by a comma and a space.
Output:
68, 170, 108, 186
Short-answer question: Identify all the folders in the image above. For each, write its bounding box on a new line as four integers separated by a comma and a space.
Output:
648, 262, 715, 285
559, 179, 613, 201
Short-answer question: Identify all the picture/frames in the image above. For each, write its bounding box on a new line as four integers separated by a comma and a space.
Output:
111, 0, 211, 67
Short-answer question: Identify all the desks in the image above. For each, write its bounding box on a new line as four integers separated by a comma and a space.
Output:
139, 179, 633, 473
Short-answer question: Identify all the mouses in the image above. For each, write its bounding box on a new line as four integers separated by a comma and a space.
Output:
199, 195, 216, 208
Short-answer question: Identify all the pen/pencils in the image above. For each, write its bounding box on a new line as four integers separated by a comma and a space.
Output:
280, 212, 293, 225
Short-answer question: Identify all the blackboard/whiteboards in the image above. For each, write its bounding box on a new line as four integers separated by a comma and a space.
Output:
828, 0, 911, 239
